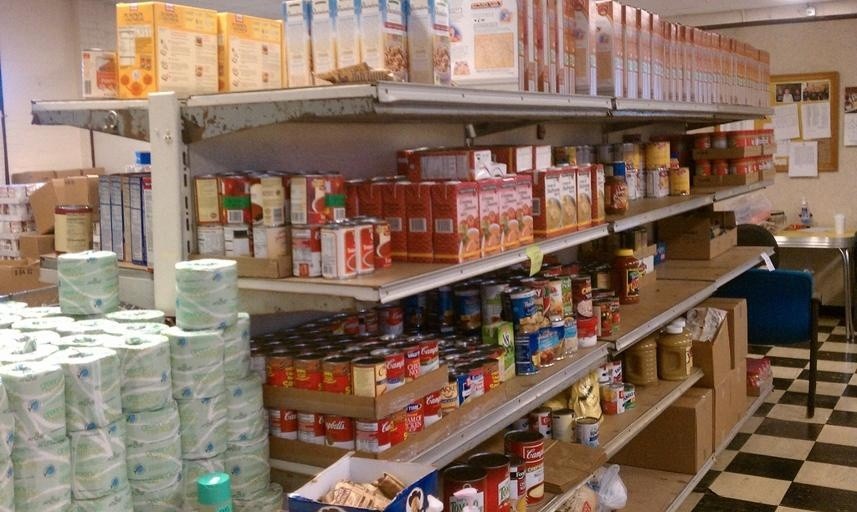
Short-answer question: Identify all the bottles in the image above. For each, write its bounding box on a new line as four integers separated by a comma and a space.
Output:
195, 471, 235, 511
834, 214, 844, 234
800, 194, 808, 222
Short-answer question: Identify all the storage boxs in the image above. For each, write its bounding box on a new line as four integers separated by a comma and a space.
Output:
0, 166, 107, 309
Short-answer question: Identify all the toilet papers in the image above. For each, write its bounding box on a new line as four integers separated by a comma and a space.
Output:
0, 249, 284, 511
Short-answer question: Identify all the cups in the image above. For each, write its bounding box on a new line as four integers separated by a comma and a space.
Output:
770, 210, 783, 237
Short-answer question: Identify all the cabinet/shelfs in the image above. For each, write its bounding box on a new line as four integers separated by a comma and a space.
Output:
27, 79, 776, 512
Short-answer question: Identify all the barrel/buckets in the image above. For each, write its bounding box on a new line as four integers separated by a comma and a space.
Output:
658, 316, 693, 380
625, 332, 657, 385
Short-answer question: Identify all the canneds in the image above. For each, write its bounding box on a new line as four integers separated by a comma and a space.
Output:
443, 360, 638, 512
250, 304, 506, 453
81, 48, 118, 99
54, 205, 93, 256
554, 130, 775, 214
191, 168, 392, 281
404, 264, 621, 375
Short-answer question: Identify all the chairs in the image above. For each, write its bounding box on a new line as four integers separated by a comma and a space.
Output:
737, 222, 780, 271
738, 225, 777, 248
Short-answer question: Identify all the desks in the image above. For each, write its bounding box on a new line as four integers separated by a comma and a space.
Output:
737, 225, 857, 344
